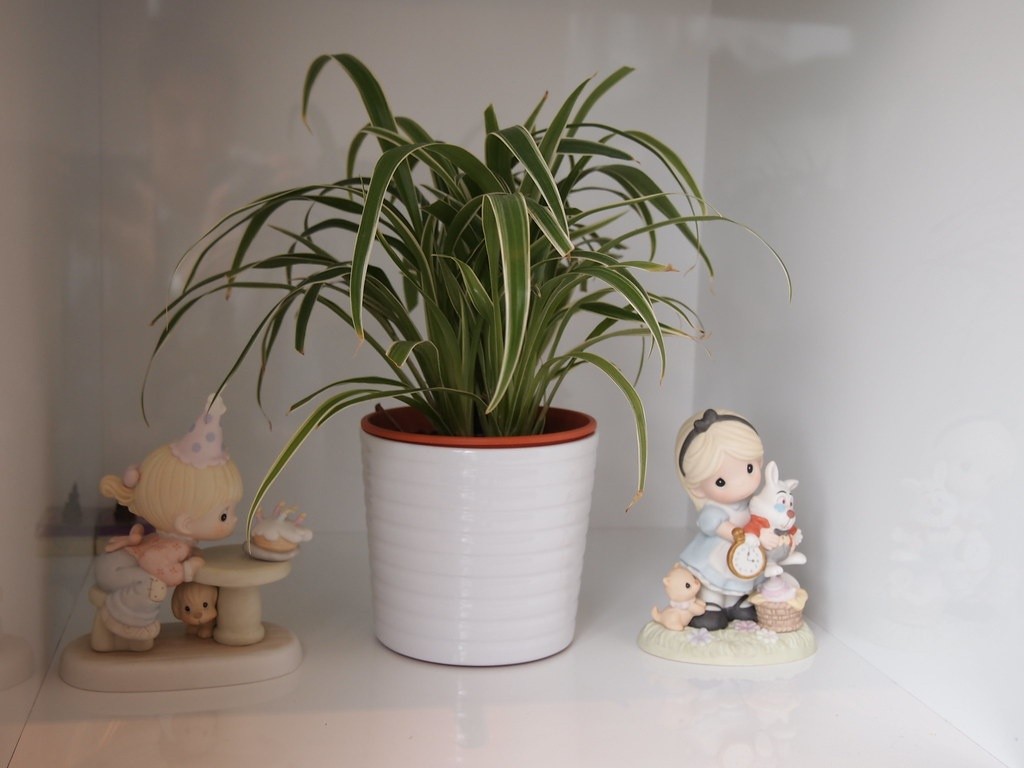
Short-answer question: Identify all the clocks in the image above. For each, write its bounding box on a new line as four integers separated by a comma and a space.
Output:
729, 527, 766, 578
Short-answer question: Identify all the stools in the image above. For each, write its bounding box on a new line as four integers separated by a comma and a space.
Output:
191, 545, 291, 645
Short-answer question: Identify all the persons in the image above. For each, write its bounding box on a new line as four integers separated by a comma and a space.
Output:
89, 392, 244, 641
674, 407, 796, 632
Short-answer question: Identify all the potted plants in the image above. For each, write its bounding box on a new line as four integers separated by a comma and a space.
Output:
141, 53, 794, 666
